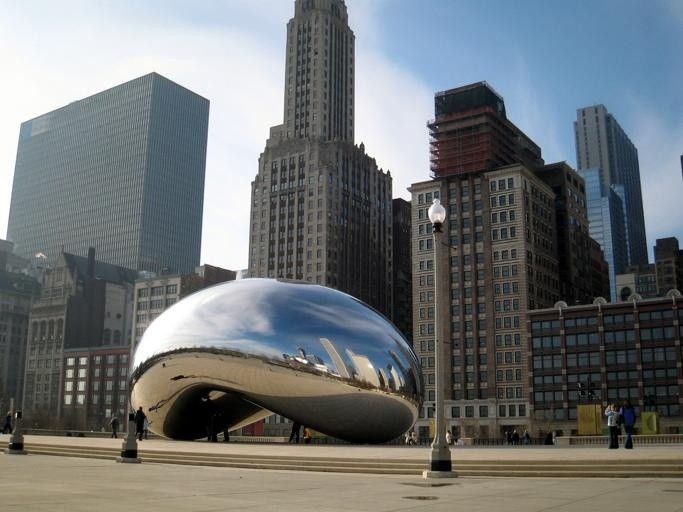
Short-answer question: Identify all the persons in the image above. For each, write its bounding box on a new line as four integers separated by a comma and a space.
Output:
402, 428, 419, 445
303, 425, 311, 444
221, 414, 230, 442
133, 406, 144, 441
148, 399, 163, 412
287, 422, 301, 443
2, 411, 12, 433
445, 430, 457, 446
502, 428, 531, 446
106, 413, 119, 438
617, 399, 636, 450
138, 416, 153, 440
210, 413, 218, 442
603, 403, 621, 449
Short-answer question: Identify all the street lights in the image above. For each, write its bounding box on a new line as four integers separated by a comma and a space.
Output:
427, 199, 452, 470
